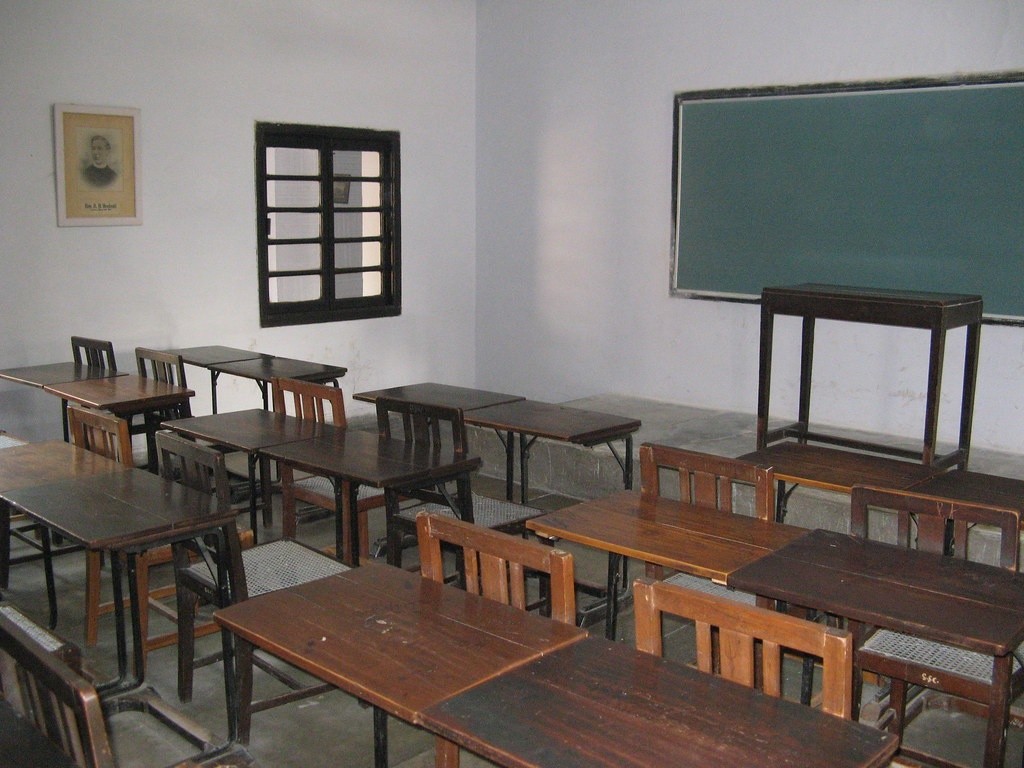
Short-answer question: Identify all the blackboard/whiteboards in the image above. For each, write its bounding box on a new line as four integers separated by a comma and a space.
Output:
666, 70, 1024, 329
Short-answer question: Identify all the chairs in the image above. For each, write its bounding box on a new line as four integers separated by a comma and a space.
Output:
0, 335, 1021, 768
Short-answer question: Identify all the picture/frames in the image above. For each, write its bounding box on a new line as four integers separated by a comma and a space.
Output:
54, 102, 142, 227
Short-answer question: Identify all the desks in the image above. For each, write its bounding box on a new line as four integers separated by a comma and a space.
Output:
735, 441, 948, 525
212, 563, 588, 768
207, 357, 348, 412
418, 637, 899, 768
44, 375, 196, 410
463, 400, 642, 540
526, 490, 810, 642
906, 470, 1024, 558
0, 439, 249, 679
0, 362, 130, 389
161, 408, 353, 539
259, 430, 481, 567
353, 382, 525, 420
727, 528, 1024, 768
756, 283, 983, 470
161, 345, 274, 414
